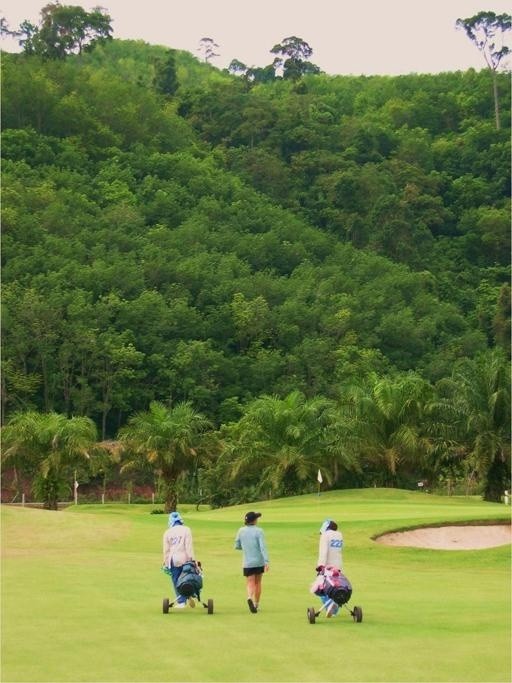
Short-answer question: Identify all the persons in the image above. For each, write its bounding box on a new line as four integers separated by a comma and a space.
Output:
315, 521, 343, 618
235, 512, 269, 613
163, 512, 195, 608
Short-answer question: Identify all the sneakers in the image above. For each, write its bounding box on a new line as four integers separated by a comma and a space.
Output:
325, 601, 337, 617
247, 598, 258, 613
176, 595, 196, 608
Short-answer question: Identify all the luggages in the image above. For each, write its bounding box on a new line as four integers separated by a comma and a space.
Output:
159, 560, 215, 614
307, 567, 363, 623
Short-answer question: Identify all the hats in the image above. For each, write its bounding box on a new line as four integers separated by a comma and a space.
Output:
245, 511, 262, 522
327, 519, 337, 530
169, 511, 183, 525
